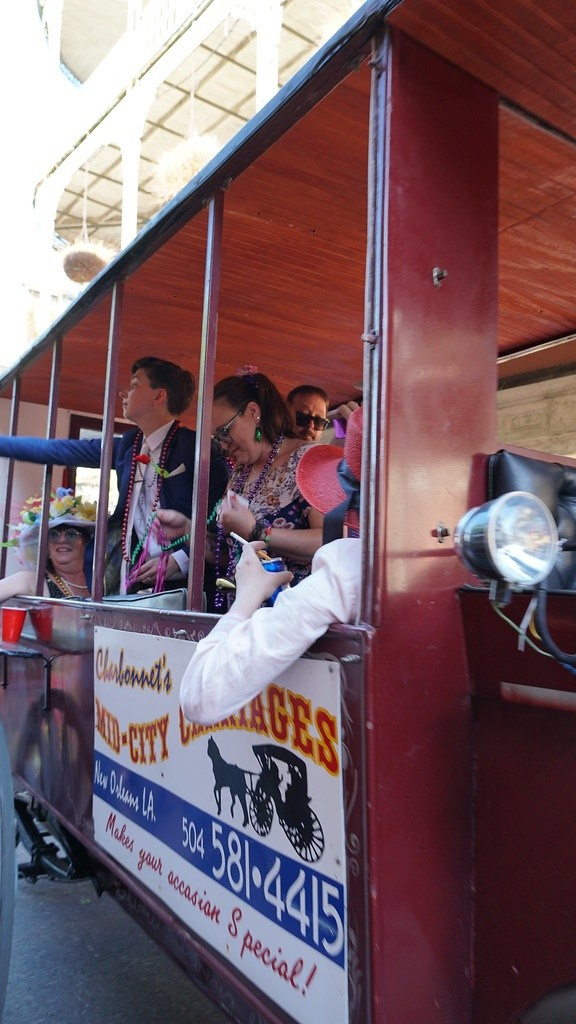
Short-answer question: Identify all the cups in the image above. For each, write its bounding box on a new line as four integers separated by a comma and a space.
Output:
0, 606, 26, 645
27, 606, 53, 644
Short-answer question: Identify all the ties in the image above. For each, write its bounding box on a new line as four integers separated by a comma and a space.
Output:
119, 441, 150, 596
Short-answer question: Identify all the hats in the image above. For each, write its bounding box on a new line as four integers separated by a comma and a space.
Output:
15, 489, 96, 572
296, 405, 363, 531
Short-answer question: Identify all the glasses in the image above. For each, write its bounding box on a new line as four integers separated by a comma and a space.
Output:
47, 528, 81, 542
294, 411, 329, 431
211, 405, 246, 446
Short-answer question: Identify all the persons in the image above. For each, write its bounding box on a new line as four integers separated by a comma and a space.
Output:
1, 355, 369, 726
